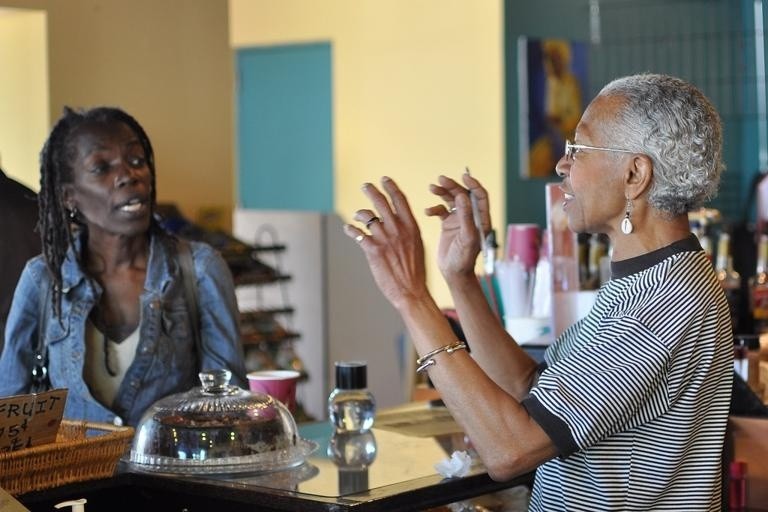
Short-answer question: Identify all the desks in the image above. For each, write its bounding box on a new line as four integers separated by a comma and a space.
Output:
119, 401, 537, 512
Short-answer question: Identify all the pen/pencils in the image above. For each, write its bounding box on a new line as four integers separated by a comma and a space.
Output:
463, 166, 488, 263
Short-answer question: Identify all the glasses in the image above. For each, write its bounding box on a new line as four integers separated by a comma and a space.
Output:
563, 137, 637, 164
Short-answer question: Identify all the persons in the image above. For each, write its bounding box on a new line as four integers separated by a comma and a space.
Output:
0, 106, 247, 452
342, 72, 733, 511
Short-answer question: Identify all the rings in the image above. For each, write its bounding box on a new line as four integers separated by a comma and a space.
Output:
352, 232, 366, 245
449, 207, 456, 213
365, 216, 380, 230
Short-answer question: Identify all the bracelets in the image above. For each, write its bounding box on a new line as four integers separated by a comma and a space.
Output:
415, 341, 468, 376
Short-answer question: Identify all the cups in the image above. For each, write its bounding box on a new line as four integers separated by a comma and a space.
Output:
246, 368, 301, 422
478, 220, 551, 319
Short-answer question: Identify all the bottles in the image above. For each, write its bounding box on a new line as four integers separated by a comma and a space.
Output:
688, 219, 768, 336
572, 229, 612, 293
733, 337, 750, 387
324, 427, 377, 499
326, 360, 379, 433
726, 461, 749, 512
